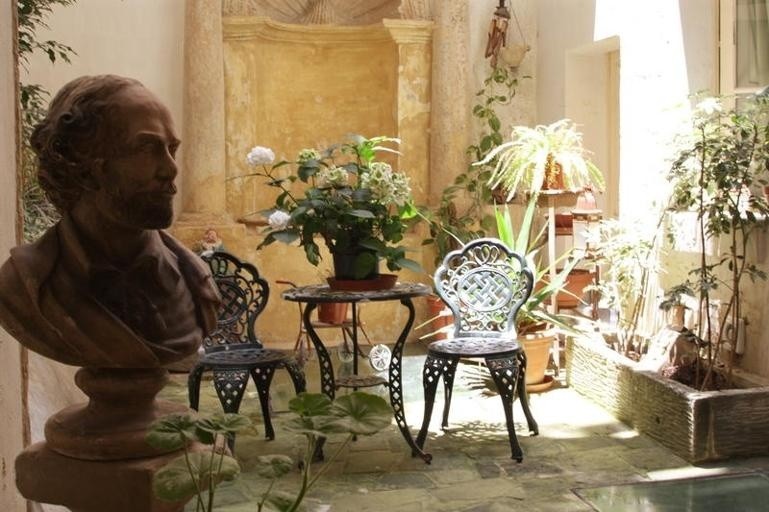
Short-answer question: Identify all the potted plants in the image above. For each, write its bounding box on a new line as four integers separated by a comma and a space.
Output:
539, 268, 592, 307
563, 83, 769, 461
415, 195, 594, 383
469, 118, 607, 202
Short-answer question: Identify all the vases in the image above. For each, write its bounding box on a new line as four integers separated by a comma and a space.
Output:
331, 249, 380, 279
317, 301, 348, 324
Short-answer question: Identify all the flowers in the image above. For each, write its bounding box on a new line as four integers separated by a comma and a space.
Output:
222, 131, 422, 280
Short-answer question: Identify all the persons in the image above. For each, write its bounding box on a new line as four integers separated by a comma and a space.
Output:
189, 226, 226, 270
0, 72, 224, 375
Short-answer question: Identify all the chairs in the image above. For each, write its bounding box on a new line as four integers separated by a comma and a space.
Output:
187, 250, 315, 440
412, 238, 540, 465
